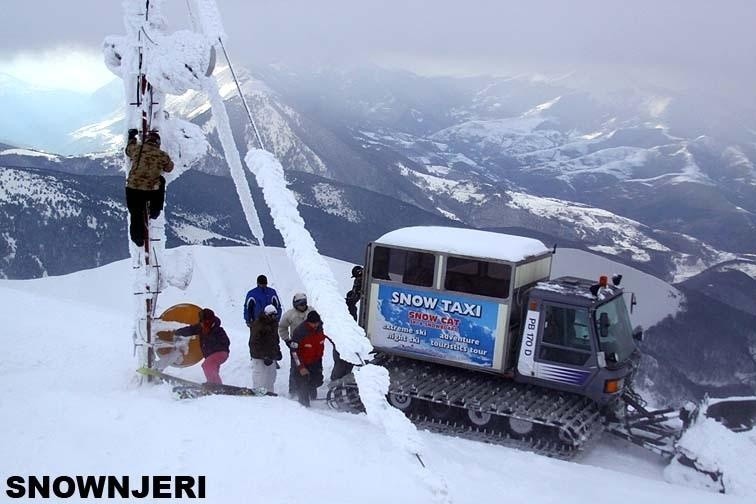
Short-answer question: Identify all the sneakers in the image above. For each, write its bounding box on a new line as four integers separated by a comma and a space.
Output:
150, 210, 160, 218
134, 240, 144, 246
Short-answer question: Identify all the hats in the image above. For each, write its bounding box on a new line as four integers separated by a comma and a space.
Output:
293, 293, 307, 308
257, 275, 267, 284
307, 311, 320, 323
199, 309, 214, 321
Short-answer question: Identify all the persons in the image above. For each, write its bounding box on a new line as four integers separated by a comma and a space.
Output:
249, 303, 284, 398
171, 308, 230, 386
123, 125, 174, 246
345, 264, 364, 322
242, 274, 283, 331
289, 308, 325, 410
278, 291, 317, 401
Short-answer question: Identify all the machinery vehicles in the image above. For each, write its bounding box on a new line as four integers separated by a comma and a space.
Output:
322, 217, 755, 497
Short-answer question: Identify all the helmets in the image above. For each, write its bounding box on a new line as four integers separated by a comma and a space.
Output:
264, 305, 278, 316
351, 266, 363, 278
145, 133, 160, 146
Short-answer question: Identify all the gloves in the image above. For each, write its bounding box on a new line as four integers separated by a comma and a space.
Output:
276, 352, 282, 360
285, 339, 291, 347
128, 129, 137, 139
264, 356, 273, 366
